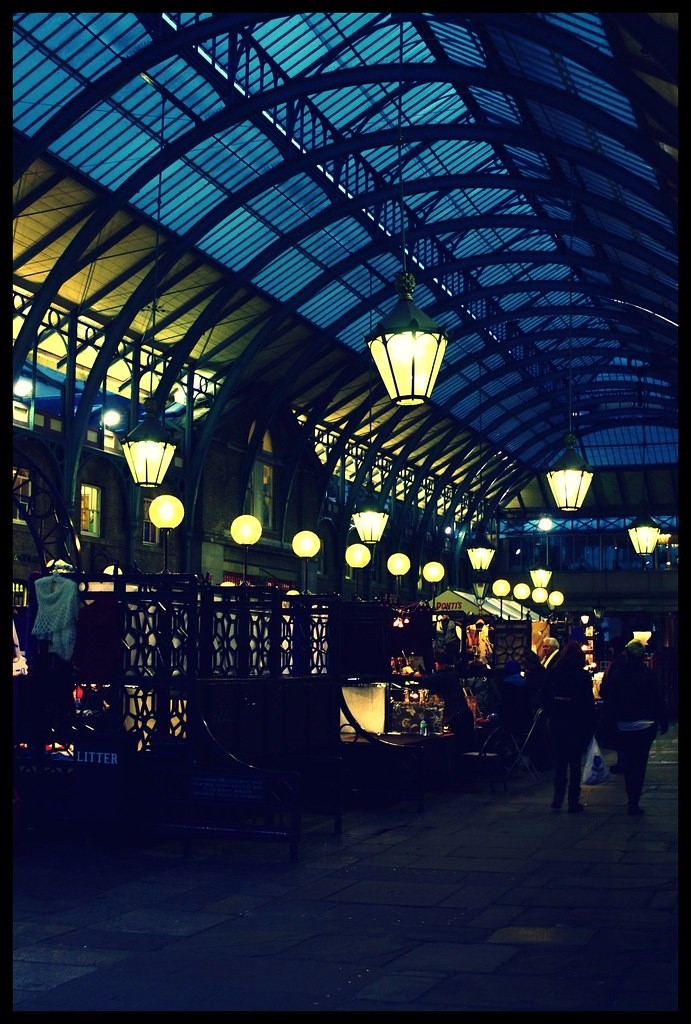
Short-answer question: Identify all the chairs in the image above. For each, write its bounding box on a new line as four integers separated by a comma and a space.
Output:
456, 725, 502, 789
500, 706, 546, 783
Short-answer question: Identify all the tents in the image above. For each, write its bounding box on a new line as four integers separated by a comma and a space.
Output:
428, 589, 549, 654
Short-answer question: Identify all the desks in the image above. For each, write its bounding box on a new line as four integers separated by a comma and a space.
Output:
339, 730, 459, 813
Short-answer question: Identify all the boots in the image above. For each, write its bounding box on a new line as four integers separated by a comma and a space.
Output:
551, 777, 568, 809
568, 785, 584, 814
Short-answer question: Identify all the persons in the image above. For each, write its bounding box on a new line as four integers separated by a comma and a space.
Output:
542, 643, 596, 812
539, 637, 560, 669
600, 637, 668, 815
418, 657, 475, 746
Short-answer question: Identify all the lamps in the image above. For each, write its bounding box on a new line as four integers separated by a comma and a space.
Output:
422, 561, 445, 585
345, 544, 372, 569
386, 553, 410, 577
529, 566, 552, 589
492, 579, 512, 597
231, 514, 262, 546
548, 591, 564, 607
513, 583, 532, 601
531, 588, 548, 603
547, 200, 594, 511
149, 495, 184, 531
365, 12, 452, 408
627, 378, 660, 556
350, 372, 391, 544
122, 94, 178, 490
292, 531, 321, 559
466, 355, 497, 572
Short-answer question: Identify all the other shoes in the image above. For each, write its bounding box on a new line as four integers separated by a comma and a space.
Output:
627, 804, 644, 815
